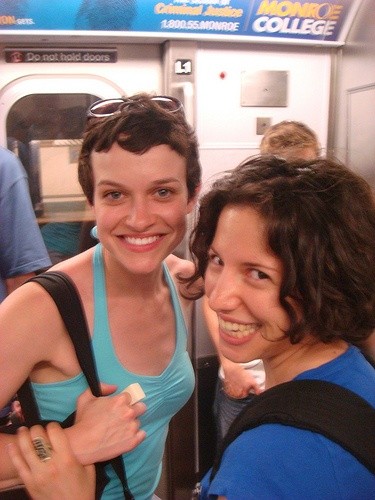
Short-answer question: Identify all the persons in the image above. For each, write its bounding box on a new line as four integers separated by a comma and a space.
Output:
1, 142, 54, 428
214, 120, 374, 459
7, 148, 375, 500
0, 91, 212, 500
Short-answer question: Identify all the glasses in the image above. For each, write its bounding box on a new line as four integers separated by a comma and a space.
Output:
86, 95, 185, 124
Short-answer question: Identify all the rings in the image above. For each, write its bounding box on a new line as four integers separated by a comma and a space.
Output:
32, 437, 54, 463
